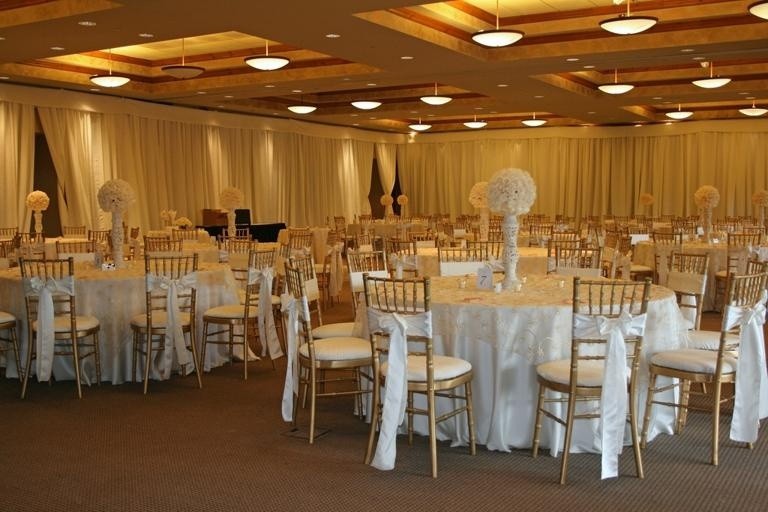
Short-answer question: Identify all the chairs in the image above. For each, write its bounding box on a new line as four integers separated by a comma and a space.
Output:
128, 249, 206, 396
287, 249, 359, 407
531, 273, 656, 487
1, 170, 768, 337
18, 254, 104, 400
359, 271, 479, 480
0, 309, 25, 390
200, 246, 277, 380
641, 270, 767, 467
664, 271, 711, 430
280, 259, 379, 444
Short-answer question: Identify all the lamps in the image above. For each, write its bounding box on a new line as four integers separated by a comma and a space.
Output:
691, 62, 732, 88
243, 40, 291, 72
663, 104, 694, 121
419, 82, 453, 106
463, 115, 488, 129
409, 117, 433, 131
471, 0, 525, 51
88, 48, 132, 90
161, 38, 206, 81
350, 90, 382, 110
520, 113, 548, 127
738, 99, 766, 118
748, 0, 767, 25
287, 94, 317, 114
599, 0, 660, 37
597, 68, 635, 95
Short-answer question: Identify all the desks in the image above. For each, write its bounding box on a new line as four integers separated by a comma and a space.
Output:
11, 250, 234, 388
364, 269, 677, 459
